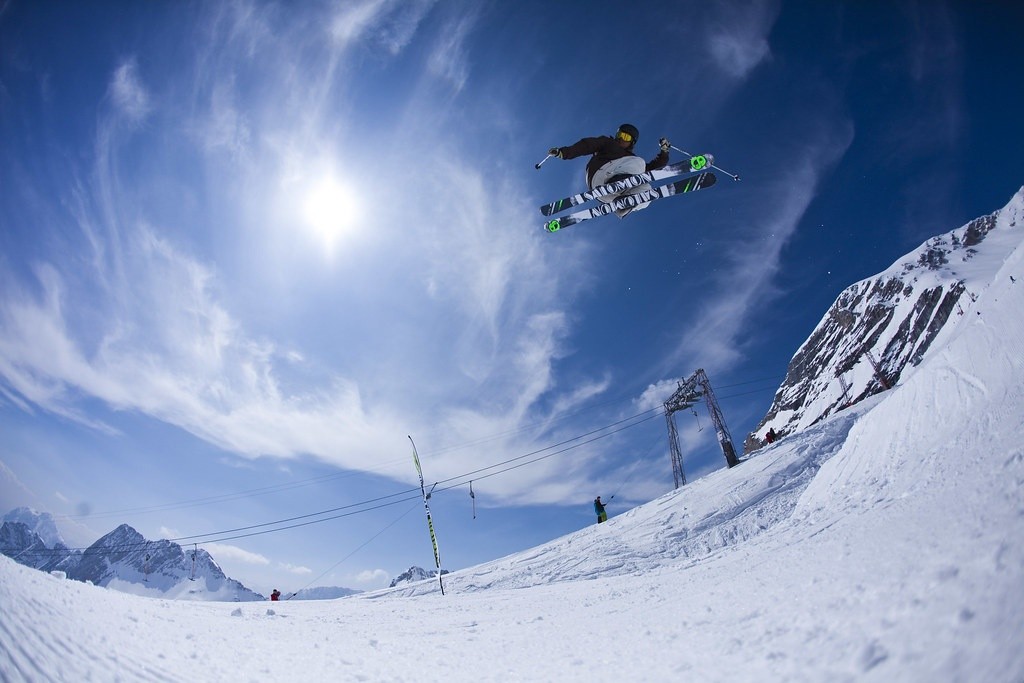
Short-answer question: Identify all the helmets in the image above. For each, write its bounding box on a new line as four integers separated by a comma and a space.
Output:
618, 124, 639, 143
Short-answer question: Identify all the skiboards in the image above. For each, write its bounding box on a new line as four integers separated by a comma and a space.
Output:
539, 151, 716, 218
543, 172, 717, 233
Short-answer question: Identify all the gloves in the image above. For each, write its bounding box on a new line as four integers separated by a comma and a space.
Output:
659, 137, 671, 151
548, 147, 563, 159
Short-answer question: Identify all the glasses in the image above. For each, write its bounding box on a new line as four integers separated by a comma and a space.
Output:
616, 132, 633, 143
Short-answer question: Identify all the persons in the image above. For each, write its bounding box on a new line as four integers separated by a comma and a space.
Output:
961, 310, 963, 315
549, 123, 669, 219
977, 312, 980, 315
1010, 276, 1015, 283
766, 428, 776, 444
271, 589, 281, 601
972, 299, 975, 302
594, 496, 607, 524
